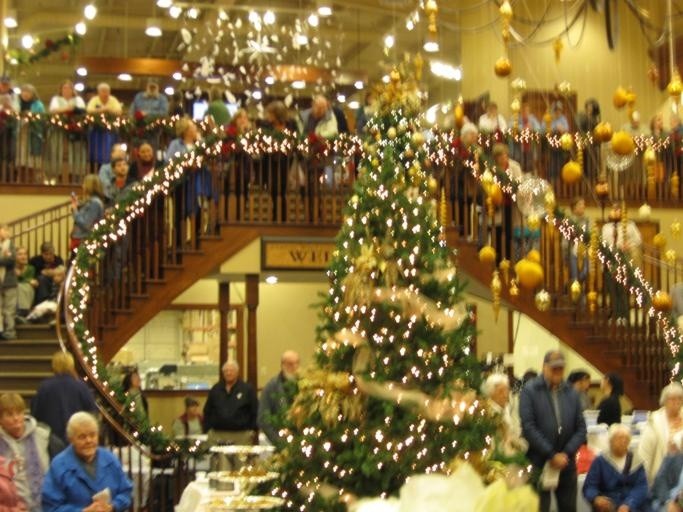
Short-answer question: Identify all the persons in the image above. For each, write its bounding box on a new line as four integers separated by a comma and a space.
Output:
171, 398, 202, 440
203, 358, 254, 445
0, 393, 64, 512
41, 411, 133, 512
32, 350, 100, 442
0, 78, 682, 342
481, 348, 682, 512
256, 348, 302, 447
122, 368, 147, 426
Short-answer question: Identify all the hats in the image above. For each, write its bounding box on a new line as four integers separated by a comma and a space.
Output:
546, 351, 565, 368
